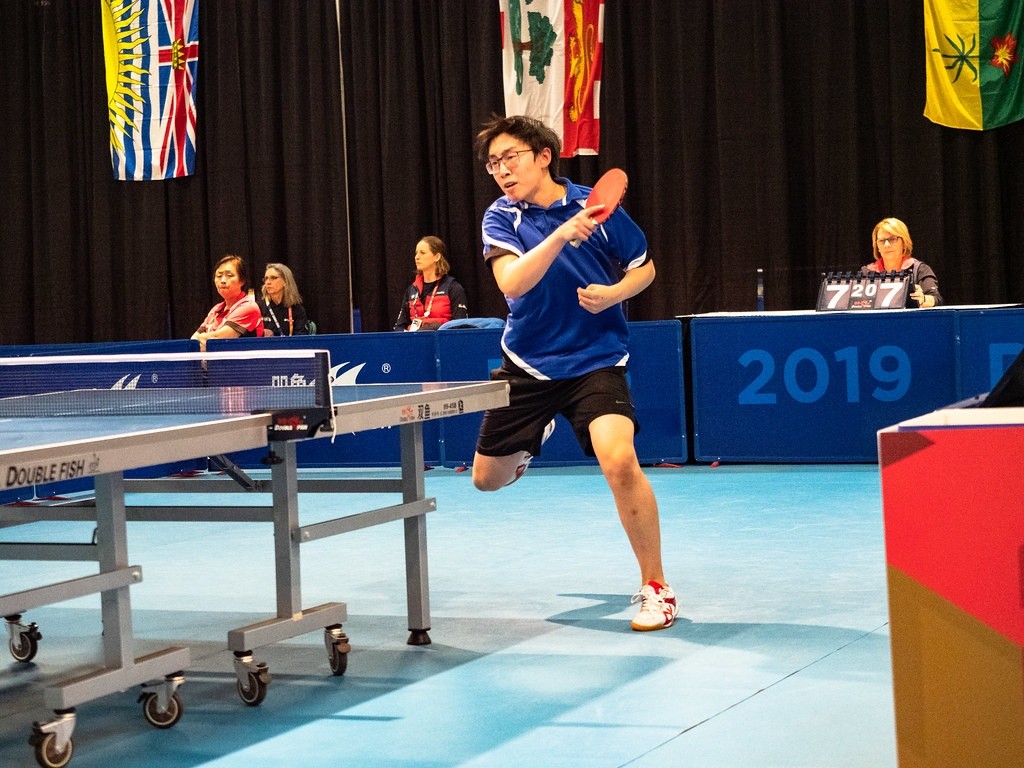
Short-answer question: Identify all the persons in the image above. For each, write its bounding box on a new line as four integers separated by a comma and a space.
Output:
255, 263, 311, 337
860, 217, 944, 310
392, 235, 469, 332
190, 255, 264, 371
472, 111, 680, 631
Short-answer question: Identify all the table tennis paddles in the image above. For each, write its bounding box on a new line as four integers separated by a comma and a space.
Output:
567, 167, 628, 250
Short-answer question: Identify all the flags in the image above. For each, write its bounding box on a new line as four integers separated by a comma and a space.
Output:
101, 0, 199, 181
923, 0, 1024, 131
499, 0, 605, 159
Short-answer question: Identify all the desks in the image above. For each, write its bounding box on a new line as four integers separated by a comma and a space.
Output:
0, 339, 205, 400
0, 356, 511, 768
205, 319, 688, 469
690, 308, 1024, 464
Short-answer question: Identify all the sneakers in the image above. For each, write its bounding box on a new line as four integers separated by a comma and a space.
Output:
630, 581, 679, 631
504, 419, 556, 486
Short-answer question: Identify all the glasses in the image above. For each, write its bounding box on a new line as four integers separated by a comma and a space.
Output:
486, 149, 533, 175
877, 235, 901, 245
262, 276, 281, 283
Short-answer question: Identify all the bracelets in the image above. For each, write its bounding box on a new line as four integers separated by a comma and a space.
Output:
920, 295, 926, 305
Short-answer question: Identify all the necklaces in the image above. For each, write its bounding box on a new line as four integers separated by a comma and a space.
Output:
207, 312, 229, 332
